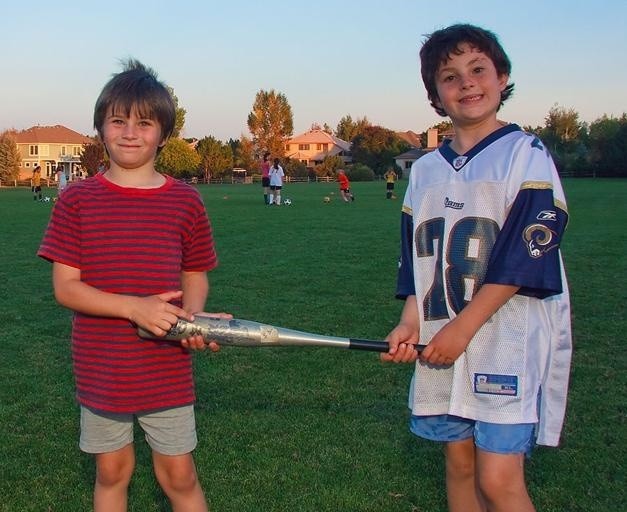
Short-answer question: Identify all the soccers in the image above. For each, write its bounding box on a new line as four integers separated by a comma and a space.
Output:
284, 199, 292, 206
323, 196, 329, 202
52, 196, 59, 202
43, 196, 50, 202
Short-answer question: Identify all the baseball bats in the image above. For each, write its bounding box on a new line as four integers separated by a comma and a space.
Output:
138, 311, 427, 355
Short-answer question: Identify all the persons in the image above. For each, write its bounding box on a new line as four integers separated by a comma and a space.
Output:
379, 24, 572, 512
31, 166, 67, 202
383, 166, 397, 199
334, 169, 354, 202
260, 152, 285, 206
36, 60, 232, 512
